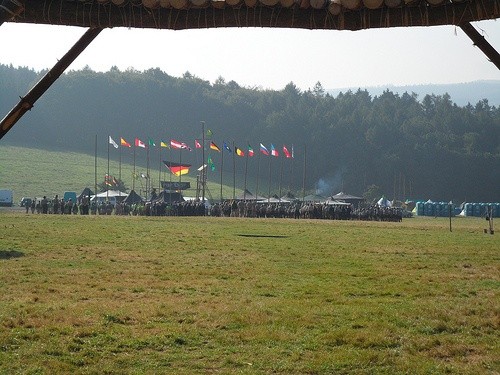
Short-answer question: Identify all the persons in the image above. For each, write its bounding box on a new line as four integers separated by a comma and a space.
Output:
24, 195, 403, 222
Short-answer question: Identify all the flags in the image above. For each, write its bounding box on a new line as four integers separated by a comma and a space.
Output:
271, 145, 279, 157
195, 138, 202, 149
135, 138, 147, 149
248, 145, 254, 156
149, 137, 156, 147
292, 147, 296, 159
283, 146, 291, 158
121, 137, 131, 147
235, 146, 245, 156
210, 141, 221, 152
108, 136, 119, 149
171, 139, 192, 152
259, 144, 270, 156
163, 161, 192, 177
224, 142, 232, 153
161, 138, 169, 148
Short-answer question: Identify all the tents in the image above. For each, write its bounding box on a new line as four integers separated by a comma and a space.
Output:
124, 190, 144, 203
90, 189, 129, 198
224, 189, 364, 205
